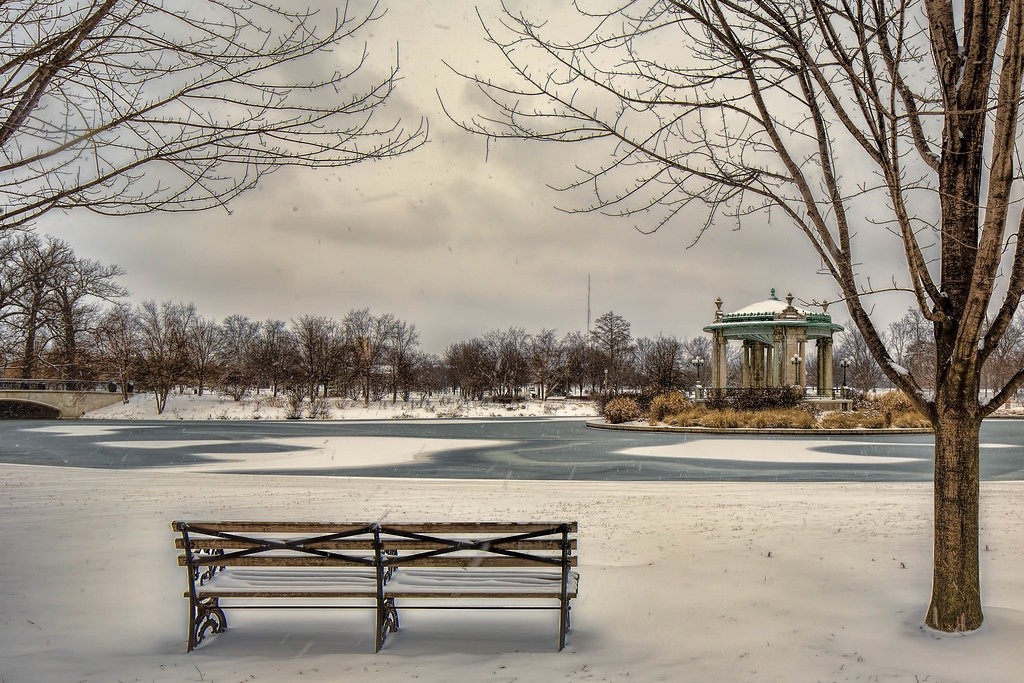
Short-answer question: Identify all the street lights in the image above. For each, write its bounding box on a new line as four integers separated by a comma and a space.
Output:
604, 369, 609, 399
691, 356, 704, 385
790, 353, 802, 385
839, 358, 851, 386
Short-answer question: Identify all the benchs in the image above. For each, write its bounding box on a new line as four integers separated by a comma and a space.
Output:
173, 521, 580, 652
